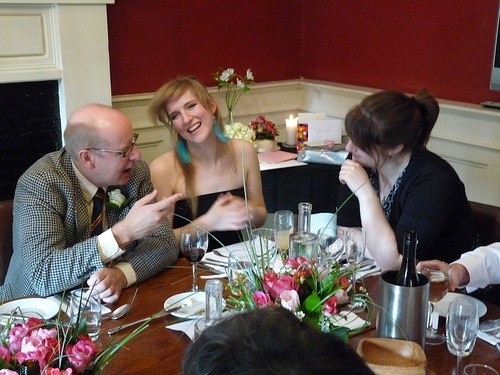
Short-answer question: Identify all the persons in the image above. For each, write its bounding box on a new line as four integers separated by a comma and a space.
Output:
183, 305, 376, 375
148, 76, 266, 254
417, 241, 500, 293
329, 90, 494, 299
0, 103, 183, 304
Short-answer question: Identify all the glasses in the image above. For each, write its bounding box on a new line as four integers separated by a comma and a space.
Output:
84, 133, 139, 158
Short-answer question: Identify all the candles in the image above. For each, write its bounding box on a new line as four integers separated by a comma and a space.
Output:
285, 113, 298, 145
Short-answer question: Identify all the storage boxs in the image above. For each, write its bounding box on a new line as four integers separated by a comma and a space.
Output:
299, 114, 342, 147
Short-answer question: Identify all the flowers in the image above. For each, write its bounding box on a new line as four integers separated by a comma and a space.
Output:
214, 67, 278, 144
105, 189, 132, 217
227, 232, 373, 337
0, 304, 151, 375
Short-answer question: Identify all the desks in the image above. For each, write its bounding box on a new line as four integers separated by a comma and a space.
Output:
0, 251, 500, 375
260, 154, 339, 221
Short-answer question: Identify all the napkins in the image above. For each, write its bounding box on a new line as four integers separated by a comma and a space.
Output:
163, 320, 202, 341
325, 309, 370, 330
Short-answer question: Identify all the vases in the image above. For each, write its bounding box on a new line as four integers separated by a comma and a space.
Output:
255, 139, 274, 153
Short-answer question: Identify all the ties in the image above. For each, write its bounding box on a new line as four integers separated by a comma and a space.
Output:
91, 188, 106, 236
